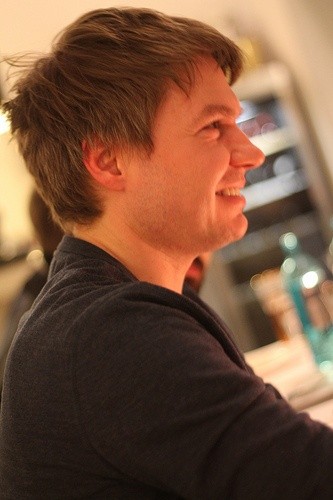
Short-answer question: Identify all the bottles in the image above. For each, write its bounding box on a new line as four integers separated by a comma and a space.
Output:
279, 231, 333, 384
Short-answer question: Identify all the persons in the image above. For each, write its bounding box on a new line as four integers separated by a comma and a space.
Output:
0, 8, 332, 500
1, 185, 209, 403
